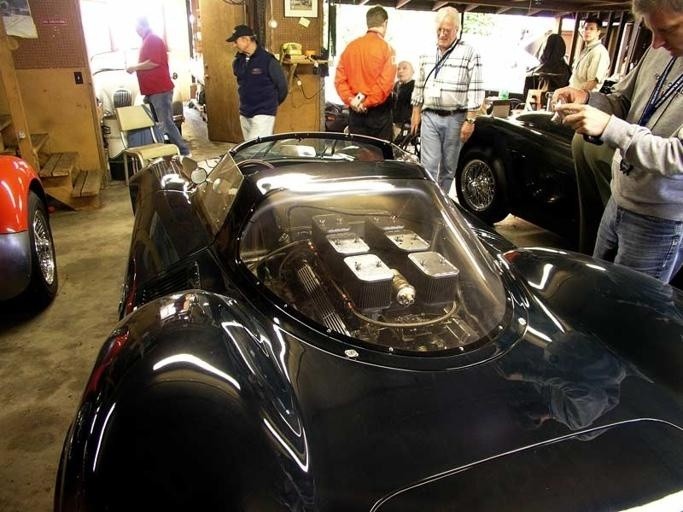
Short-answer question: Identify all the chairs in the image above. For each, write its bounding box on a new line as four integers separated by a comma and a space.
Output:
116, 104, 179, 186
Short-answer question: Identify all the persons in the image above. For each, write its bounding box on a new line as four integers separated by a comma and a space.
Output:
556, 1, 683, 285
569, 16, 611, 106
501, 330, 626, 442
335, 7, 396, 160
228, 26, 288, 143
569, 131, 615, 255
410, 5, 486, 194
394, 61, 415, 125
125, 19, 191, 157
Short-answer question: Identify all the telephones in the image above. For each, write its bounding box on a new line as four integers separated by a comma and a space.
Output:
282, 42, 302, 56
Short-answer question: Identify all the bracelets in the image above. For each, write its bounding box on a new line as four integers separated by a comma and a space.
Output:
465, 118, 477, 125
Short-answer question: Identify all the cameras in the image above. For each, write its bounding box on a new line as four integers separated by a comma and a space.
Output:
549, 98, 572, 125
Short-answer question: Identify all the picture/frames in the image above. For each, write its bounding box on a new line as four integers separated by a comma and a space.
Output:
282, 0, 319, 18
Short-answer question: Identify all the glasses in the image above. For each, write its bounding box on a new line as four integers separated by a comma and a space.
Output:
435, 26, 457, 34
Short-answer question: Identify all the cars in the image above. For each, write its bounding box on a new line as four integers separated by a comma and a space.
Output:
89, 49, 184, 134
53, 133, 683, 506
456, 112, 682, 286
0, 154, 58, 318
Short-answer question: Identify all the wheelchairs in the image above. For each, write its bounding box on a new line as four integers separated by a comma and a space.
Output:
392, 107, 420, 159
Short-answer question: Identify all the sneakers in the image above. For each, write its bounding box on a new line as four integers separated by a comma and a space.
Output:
225, 23, 255, 43
